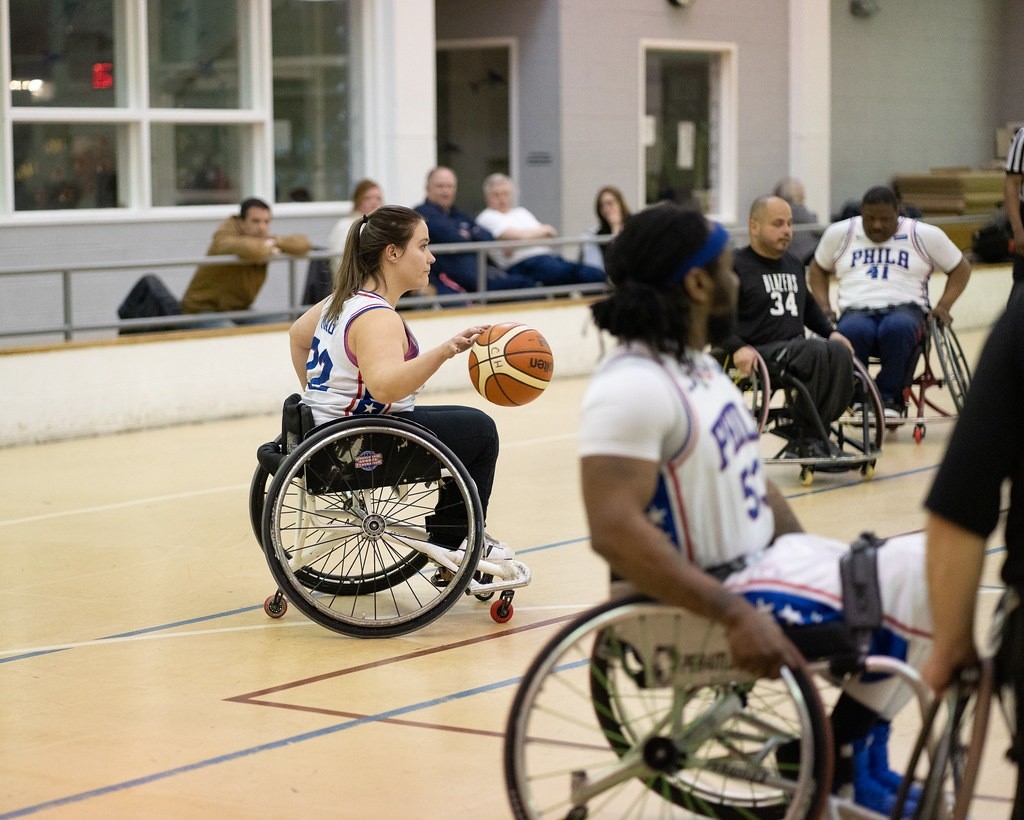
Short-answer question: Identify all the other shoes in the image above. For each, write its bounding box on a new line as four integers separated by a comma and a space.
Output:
796, 431, 840, 463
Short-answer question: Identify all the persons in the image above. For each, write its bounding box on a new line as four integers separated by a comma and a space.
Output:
581, 185, 631, 289
328, 180, 382, 290
1004, 125, 1024, 257
579, 198, 932, 797
918, 277, 1024, 819
777, 176, 820, 263
474, 170, 607, 297
708, 194, 861, 471
415, 166, 539, 306
809, 185, 971, 430
181, 199, 310, 328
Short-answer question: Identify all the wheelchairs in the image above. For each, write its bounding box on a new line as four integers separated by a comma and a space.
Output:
706, 341, 885, 485
809, 317, 972, 444
500, 546, 1024, 820
249, 394, 531, 638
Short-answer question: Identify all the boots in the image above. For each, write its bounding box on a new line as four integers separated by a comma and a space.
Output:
832, 733, 918, 819
870, 730, 924, 802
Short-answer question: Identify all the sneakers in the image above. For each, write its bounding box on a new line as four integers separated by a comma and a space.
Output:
882, 395, 902, 419
428, 528, 515, 564
848, 392, 875, 419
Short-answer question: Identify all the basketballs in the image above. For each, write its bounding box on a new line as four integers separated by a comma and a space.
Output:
467, 322, 556, 408
